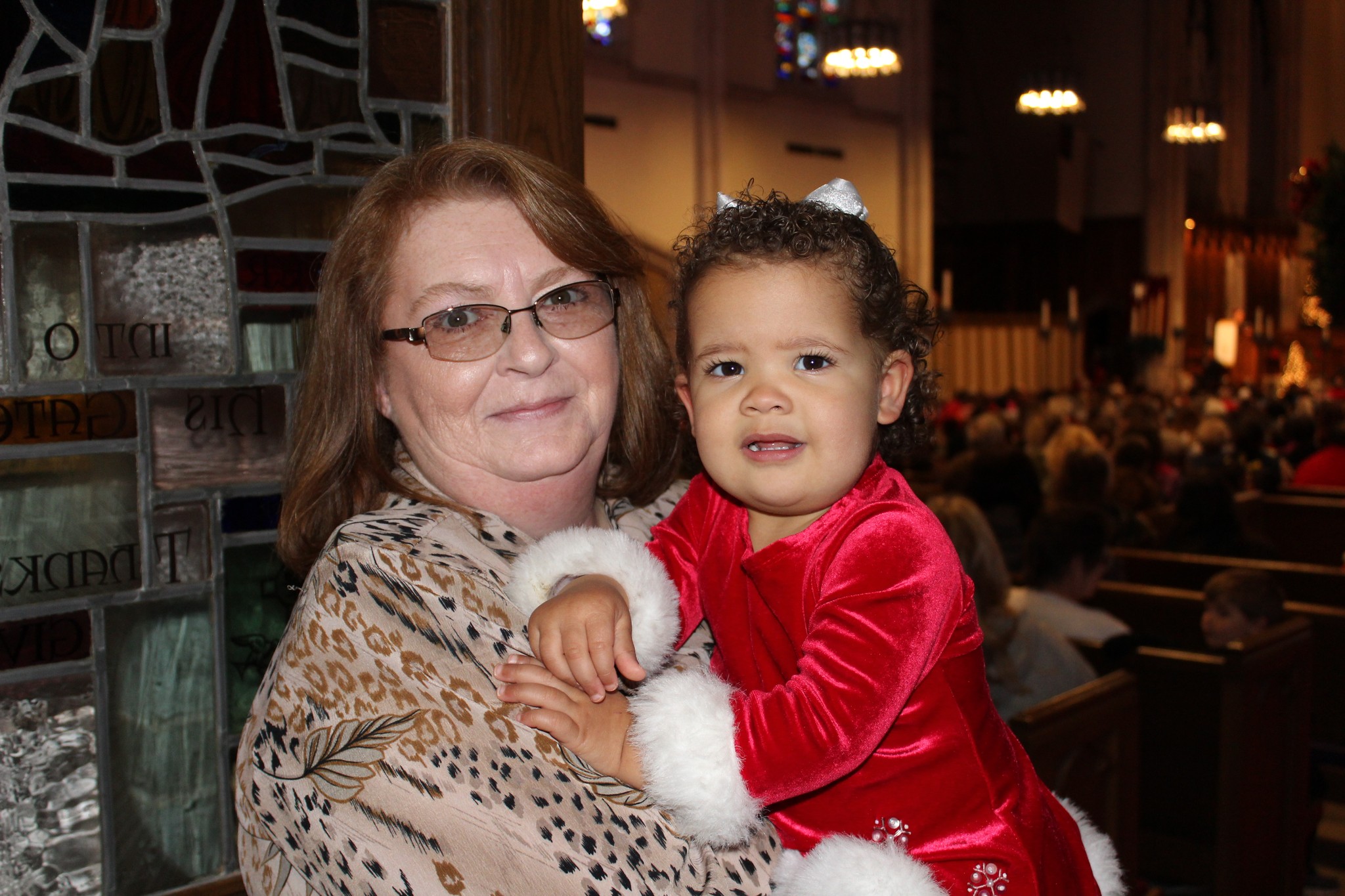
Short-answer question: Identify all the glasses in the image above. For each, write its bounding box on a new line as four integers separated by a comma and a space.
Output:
378, 281, 617, 363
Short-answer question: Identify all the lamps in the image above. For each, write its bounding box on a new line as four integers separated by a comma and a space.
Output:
1161, 0, 1227, 146
820, 0, 903, 78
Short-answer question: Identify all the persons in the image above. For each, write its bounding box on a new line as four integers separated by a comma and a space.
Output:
233, 140, 786, 896
1199, 567, 1286, 654
948, 320, 1345, 600
493, 177, 1132, 896
927, 495, 1140, 719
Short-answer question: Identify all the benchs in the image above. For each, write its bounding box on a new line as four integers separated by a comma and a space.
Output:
1006, 493, 1345, 896
